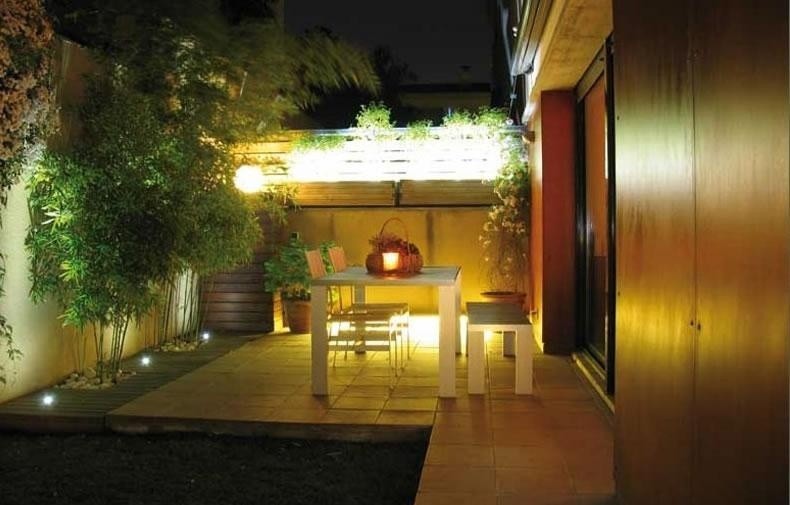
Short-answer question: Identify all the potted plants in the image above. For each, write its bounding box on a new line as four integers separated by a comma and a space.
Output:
265, 238, 342, 334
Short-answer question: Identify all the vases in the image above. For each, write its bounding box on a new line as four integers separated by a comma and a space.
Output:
481, 290, 526, 314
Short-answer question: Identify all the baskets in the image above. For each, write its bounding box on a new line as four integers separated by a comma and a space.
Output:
366, 218, 423, 276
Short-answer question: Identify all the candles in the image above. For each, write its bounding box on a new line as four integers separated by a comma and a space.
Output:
382, 252, 399, 271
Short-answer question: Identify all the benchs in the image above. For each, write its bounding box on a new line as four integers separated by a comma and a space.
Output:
466, 302, 532, 395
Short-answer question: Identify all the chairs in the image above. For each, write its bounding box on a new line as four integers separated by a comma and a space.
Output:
326, 244, 409, 370
305, 247, 399, 389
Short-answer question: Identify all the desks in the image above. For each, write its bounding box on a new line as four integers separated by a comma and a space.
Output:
311, 266, 463, 398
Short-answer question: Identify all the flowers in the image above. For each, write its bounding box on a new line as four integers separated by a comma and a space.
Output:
478, 149, 531, 294
